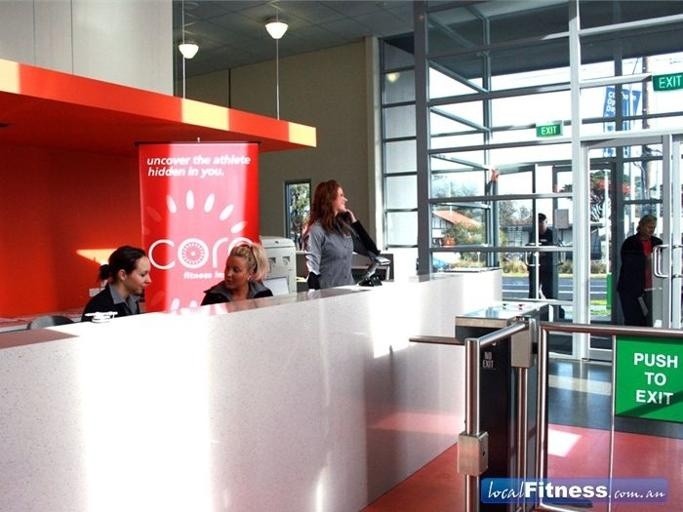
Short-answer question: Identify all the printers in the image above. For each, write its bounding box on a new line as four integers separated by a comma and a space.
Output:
259, 235, 297, 297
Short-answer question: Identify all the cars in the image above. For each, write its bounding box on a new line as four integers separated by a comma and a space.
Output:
430, 251, 462, 271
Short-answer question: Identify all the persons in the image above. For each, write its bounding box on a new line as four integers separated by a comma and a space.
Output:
616, 213, 665, 327
526, 212, 566, 320
199, 243, 274, 307
77, 244, 151, 321
304, 177, 382, 291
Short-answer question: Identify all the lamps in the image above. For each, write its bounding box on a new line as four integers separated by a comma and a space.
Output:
262, 17, 289, 41
176, 40, 200, 61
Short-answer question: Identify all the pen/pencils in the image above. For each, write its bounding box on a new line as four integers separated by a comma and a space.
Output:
84, 311, 118, 317
